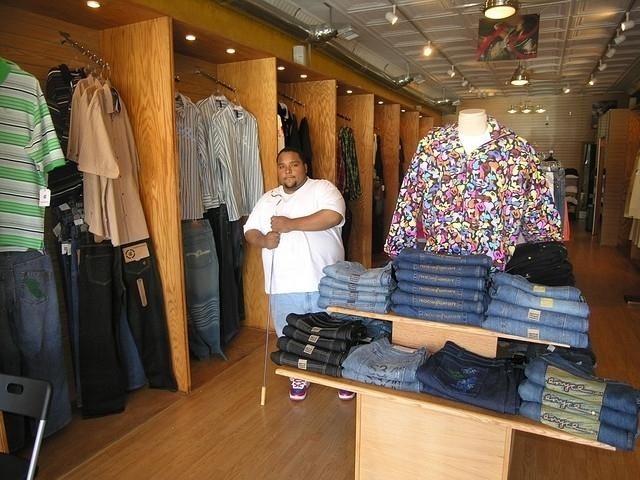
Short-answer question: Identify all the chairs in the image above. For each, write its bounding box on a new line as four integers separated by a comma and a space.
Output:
0, 374, 56, 480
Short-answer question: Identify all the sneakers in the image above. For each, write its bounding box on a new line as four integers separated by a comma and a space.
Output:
337, 390, 355, 400
288, 377, 310, 401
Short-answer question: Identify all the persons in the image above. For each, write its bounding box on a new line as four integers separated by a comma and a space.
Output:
243, 148, 357, 402
542, 150, 565, 212
383, 105, 562, 272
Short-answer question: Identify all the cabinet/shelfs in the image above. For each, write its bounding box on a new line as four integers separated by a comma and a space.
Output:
565, 174, 580, 222
590, 139, 626, 247
275, 304, 616, 479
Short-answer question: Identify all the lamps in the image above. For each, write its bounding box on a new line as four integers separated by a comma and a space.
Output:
483, 0, 519, 19
507, 100, 544, 116
421, 40, 433, 58
391, 59, 413, 88
304, 9, 337, 47
447, 63, 476, 95
584, 10, 636, 88
510, 60, 529, 88
561, 83, 571, 97
384, 3, 400, 28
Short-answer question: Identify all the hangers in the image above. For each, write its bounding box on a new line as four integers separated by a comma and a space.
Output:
210, 75, 241, 112
337, 110, 351, 126
56, 199, 91, 236
61, 39, 112, 90
281, 93, 307, 116
